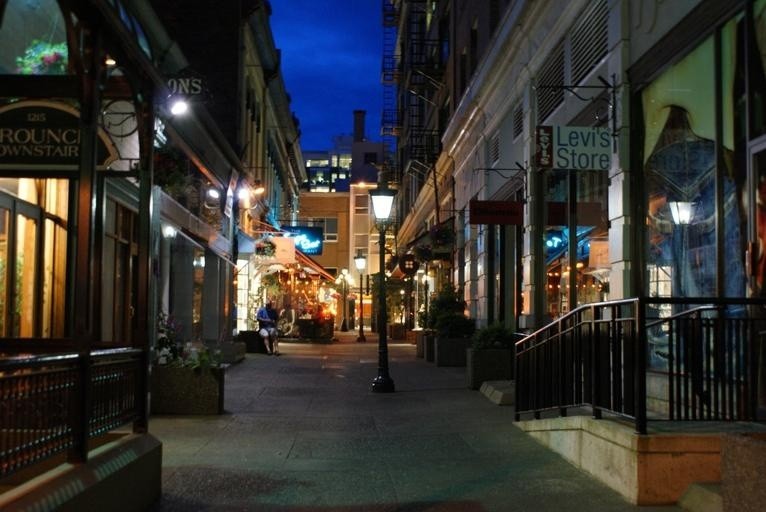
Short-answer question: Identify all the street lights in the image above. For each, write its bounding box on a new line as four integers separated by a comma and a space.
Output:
360, 166, 396, 394
340, 268, 348, 332
353, 247, 365, 342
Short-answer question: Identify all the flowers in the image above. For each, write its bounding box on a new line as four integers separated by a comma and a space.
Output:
149, 309, 224, 377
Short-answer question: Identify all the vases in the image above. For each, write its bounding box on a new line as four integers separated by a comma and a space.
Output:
150, 363, 231, 415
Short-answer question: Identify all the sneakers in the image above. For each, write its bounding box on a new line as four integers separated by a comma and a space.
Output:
268, 350, 281, 356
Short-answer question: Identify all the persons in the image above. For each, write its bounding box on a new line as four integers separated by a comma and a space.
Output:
255, 300, 281, 356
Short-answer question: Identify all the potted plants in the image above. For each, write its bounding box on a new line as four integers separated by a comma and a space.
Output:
466, 319, 514, 390
416, 282, 476, 367
385, 276, 417, 341
